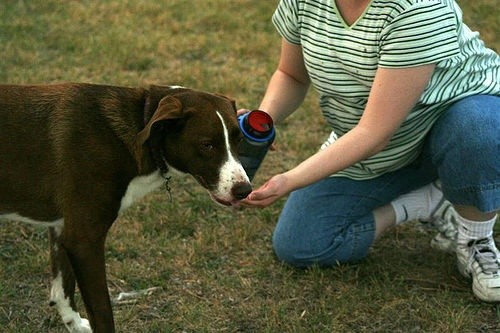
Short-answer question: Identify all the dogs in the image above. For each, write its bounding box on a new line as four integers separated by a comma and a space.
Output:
0, 82, 252, 333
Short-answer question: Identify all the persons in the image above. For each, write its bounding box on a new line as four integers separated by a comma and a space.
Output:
237, 0, 500, 303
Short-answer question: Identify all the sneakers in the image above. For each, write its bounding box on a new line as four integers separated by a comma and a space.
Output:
456, 234, 500, 302
418, 179, 458, 250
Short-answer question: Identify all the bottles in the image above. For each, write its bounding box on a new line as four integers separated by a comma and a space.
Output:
232, 109, 275, 183
